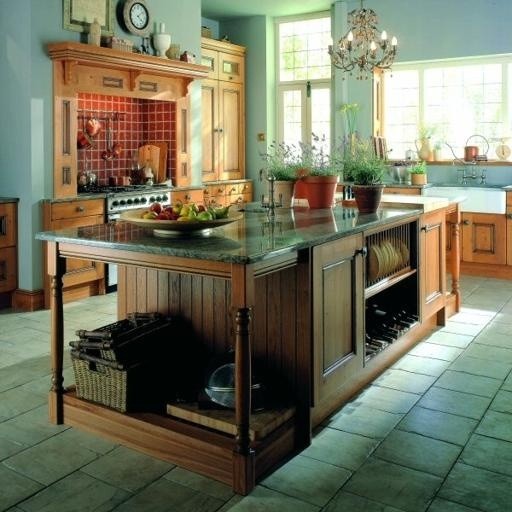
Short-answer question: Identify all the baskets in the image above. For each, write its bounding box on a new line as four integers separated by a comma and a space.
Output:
69, 311, 176, 414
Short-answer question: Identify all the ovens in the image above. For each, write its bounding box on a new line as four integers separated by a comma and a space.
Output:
106, 214, 124, 293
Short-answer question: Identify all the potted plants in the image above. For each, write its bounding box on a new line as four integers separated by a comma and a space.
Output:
415, 123, 442, 162
405, 158, 428, 185
257, 140, 303, 208
339, 141, 393, 215
298, 132, 340, 208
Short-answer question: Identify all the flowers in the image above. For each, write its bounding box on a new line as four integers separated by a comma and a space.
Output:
340, 102, 362, 137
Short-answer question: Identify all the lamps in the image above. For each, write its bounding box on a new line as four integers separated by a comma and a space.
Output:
327, 0, 397, 81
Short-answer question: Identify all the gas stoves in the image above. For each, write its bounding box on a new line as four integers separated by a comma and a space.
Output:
91, 185, 172, 221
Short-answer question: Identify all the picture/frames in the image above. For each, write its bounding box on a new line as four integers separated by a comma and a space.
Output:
62, 0, 116, 38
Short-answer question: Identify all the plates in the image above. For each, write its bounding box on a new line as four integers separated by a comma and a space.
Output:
368, 239, 411, 282
121, 209, 243, 235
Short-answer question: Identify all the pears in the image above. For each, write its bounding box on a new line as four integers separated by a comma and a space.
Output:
140, 200, 232, 222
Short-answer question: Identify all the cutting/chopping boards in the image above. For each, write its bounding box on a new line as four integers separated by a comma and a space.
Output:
137, 144, 160, 185
143, 142, 168, 183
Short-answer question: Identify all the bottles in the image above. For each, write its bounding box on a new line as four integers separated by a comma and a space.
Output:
89, 17, 102, 47
145, 167, 154, 190
390, 166, 401, 186
367, 295, 421, 351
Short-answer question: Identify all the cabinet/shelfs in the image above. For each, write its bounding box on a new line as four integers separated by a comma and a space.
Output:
0, 197, 20, 293
201, 34, 253, 209
446, 211, 512, 280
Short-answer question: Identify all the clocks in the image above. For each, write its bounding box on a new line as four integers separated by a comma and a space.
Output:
122, 1, 152, 36
